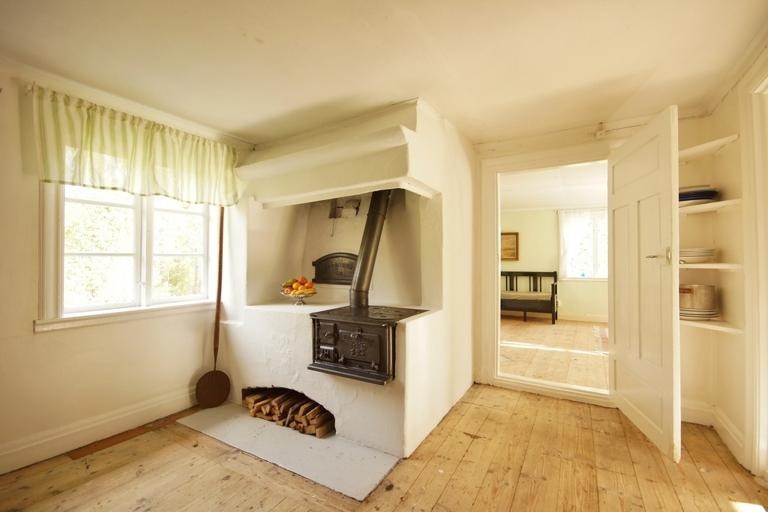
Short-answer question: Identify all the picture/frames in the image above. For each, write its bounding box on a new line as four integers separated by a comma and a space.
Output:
500, 232, 519, 262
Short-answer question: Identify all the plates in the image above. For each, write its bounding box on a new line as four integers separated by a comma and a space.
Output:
678, 183, 721, 208
680, 309, 721, 321
678, 247, 717, 263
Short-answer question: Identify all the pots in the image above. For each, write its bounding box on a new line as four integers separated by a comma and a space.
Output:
679, 284, 717, 310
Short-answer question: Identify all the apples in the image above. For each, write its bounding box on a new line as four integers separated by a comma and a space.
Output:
284, 276, 313, 294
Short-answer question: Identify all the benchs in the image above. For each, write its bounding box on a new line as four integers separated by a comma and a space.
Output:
500, 269, 560, 324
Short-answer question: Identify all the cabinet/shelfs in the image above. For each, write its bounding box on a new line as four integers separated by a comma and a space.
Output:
676, 134, 747, 335
